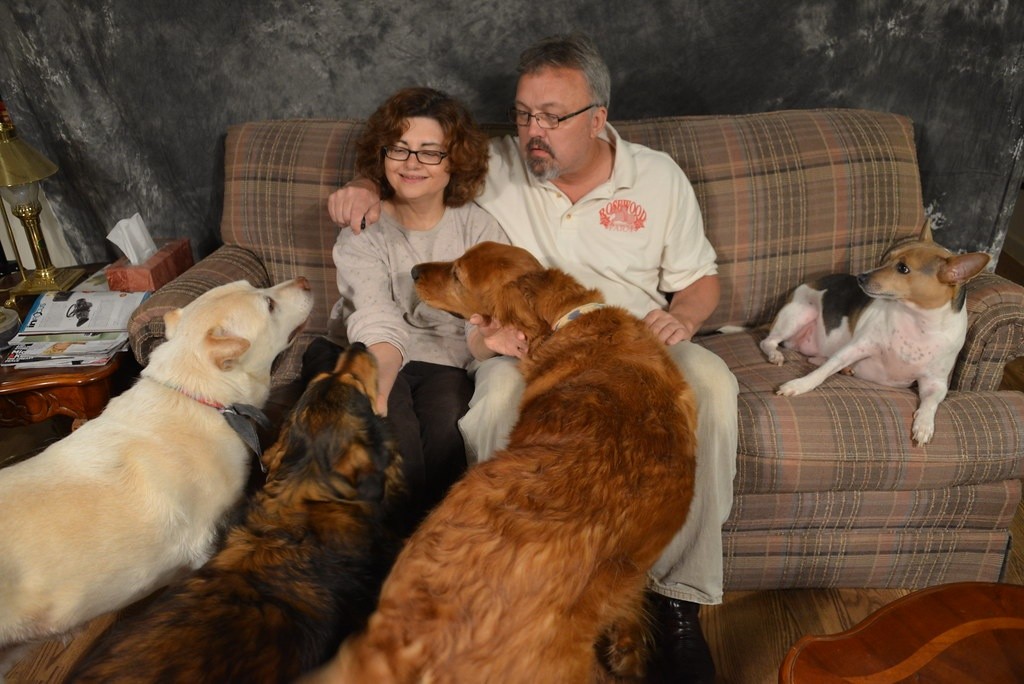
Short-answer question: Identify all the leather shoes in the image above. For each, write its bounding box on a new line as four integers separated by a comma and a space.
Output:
646, 592, 716, 684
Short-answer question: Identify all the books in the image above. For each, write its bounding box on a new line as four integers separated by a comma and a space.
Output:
1, 291, 151, 369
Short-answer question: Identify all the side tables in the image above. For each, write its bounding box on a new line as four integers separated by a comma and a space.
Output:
0, 260, 130, 433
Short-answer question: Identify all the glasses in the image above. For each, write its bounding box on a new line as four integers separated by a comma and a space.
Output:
508, 104, 602, 129
382, 145, 450, 165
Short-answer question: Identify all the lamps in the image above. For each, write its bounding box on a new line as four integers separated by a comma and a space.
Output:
0, 98, 86, 297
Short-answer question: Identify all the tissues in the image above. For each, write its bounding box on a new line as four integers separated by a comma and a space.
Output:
105, 212, 196, 292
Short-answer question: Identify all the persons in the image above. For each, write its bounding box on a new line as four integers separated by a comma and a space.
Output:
332, 87, 531, 519
328, 33, 739, 684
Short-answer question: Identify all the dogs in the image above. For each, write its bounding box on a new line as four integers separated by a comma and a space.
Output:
0, 242, 701, 684
718, 216, 993, 447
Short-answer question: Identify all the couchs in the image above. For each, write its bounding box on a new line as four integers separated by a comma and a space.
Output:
127, 111, 1024, 590
